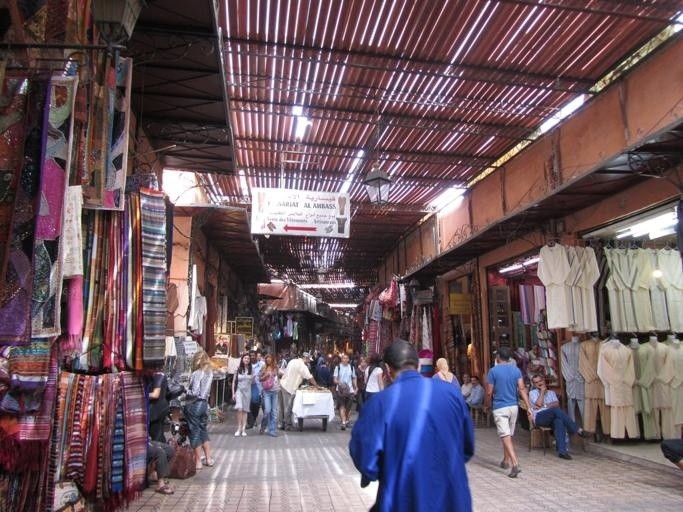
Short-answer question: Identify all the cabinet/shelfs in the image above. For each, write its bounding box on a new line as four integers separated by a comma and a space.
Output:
488, 286, 514, 368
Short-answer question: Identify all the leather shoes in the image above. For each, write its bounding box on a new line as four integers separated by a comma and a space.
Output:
559, 453, 572, 460
578, 431, 592, 438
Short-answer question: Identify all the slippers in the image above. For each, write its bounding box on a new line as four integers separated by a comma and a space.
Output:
154, 480, 175, 494
195, 467, 204, 472
203, 459, 215, 467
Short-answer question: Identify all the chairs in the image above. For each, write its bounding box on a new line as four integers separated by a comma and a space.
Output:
528, 408, 558, 456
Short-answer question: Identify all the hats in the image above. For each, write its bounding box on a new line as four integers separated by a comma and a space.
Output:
303, 352, 313, 362
338, 382, 350, 397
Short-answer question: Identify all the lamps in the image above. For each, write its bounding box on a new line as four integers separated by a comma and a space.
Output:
362, 98, 393, 205
87, 0, 144, 56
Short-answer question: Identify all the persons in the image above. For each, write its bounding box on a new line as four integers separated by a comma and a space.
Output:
660, 424, 683, 470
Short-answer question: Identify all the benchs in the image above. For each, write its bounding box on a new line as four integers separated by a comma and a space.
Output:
469, 404, 491, 427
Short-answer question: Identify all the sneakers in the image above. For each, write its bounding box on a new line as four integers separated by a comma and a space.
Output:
235, 430, 248, 437
508, 464, 521, 478
341, 420, 352, 430
501, 463, 509, 469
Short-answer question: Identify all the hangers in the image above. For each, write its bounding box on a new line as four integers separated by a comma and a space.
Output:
546, 238, 679, 251
